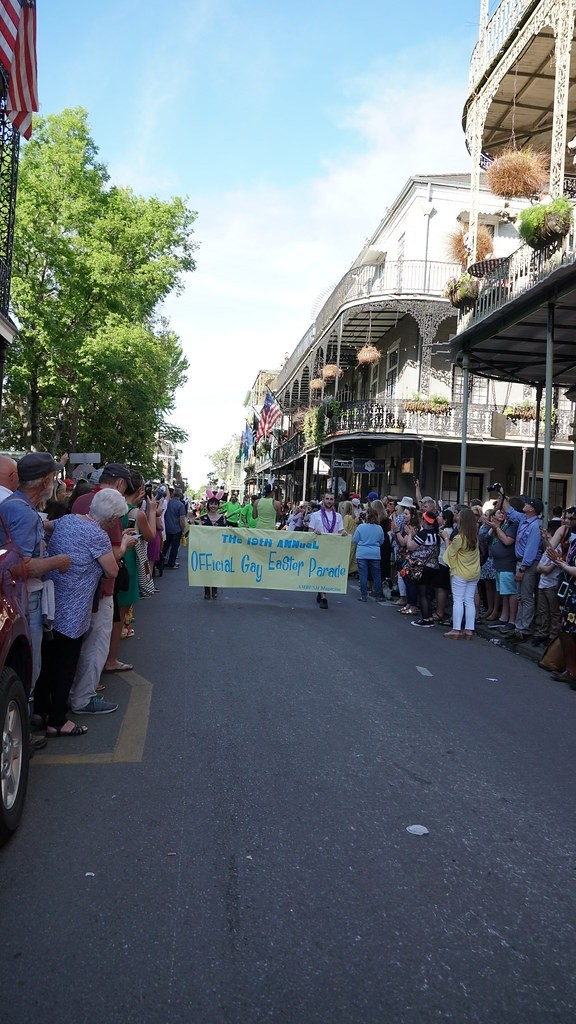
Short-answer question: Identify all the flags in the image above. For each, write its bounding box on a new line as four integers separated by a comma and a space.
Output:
239, 391, 283, 460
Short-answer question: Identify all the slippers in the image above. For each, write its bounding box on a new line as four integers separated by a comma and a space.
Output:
106, 663, 133, 671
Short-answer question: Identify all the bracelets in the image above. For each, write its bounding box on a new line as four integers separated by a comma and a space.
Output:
519, 568, 525, 573
395, 531, 400, 534
444, 537, 449, 540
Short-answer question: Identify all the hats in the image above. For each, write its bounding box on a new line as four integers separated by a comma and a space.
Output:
350, 494, 359, 499
102, 463, 135, 495
366, 492, 377, 500
397, 496, 416, 508
526, 498, 544, 514
261, 484, 274, 495
419, 496, 433, 503
18, 452, 63, 479
352, 498, 360, 506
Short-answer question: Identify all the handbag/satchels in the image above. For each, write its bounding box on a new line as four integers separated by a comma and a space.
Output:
400, 558, 424, 582
119, 559, 129, 591
134, 541, 155, 593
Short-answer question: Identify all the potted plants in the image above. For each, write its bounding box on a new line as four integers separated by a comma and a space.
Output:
445, 271, 482, 310
518, 195, 573, 252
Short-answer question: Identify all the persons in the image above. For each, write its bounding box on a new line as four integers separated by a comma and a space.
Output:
287, 479, 576, 691
189, 483, 281, 600
0, 452, 188, 760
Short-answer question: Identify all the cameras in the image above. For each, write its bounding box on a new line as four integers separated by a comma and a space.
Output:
359, 513, 365, 519
486, 482, 499, 492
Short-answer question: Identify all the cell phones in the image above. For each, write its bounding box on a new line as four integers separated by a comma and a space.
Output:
557, 581, 569, 598
131, 534, 143, 539
145, 484, 152, 499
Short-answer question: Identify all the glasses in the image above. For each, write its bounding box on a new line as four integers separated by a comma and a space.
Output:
569, 517, 576, 520
56, 480, 62, 489
389, 502, 394, 505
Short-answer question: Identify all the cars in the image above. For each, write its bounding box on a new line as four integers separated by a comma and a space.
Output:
0, 518, 34, 839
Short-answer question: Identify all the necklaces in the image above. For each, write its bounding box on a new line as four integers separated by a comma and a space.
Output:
86, 514, 96, 522
321, 506, 336, 533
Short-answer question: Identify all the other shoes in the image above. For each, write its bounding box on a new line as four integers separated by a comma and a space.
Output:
204, 589, 211, 599
317, 592, 328, 609
25, 685, 118, 749
170, 562, 180, 569
358, 597, 368, 602
393, 597, 575, 686
377, 597, 386, 603
212, 587, 217, 598
